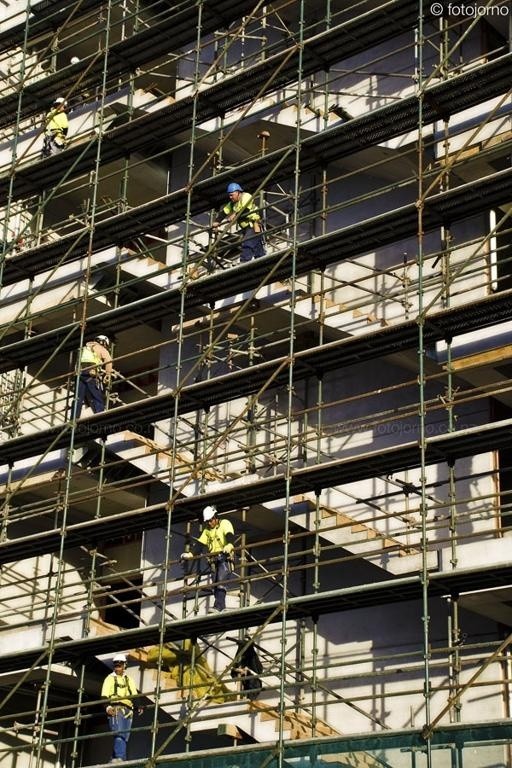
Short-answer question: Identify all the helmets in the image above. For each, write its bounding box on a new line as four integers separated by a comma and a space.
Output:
95, 334, 109, 346
112, 654, 127, 662
203, 506, 218, 522
71, 56, 79, 64
227, 183, 243, 193
54, 97, 68, 107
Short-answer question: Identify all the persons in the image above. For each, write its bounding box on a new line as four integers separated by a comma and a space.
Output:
68, 332, 110, 421
209, 180, 267, 263
185, 505, 235, 614
101, 653, 145, 764
41, 96, 71, 157
